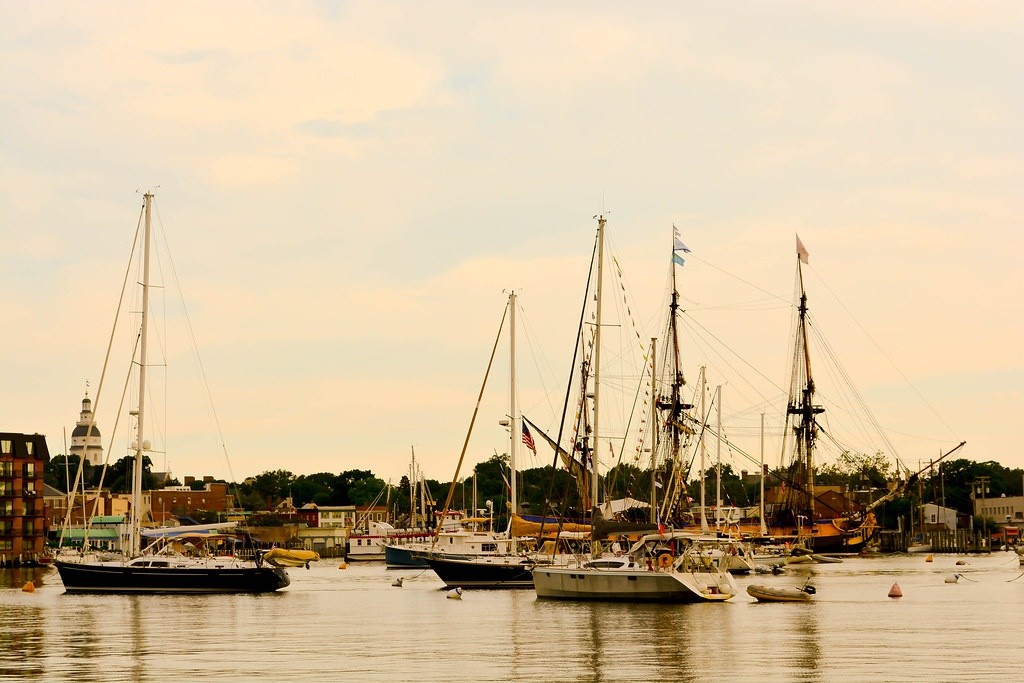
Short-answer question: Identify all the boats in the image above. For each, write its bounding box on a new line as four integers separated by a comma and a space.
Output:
689, 535, 792, 571
813, 555, 841, 562
262, 548, 312, 569
750, 584, 815, 605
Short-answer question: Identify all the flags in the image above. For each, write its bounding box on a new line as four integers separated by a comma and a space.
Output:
671, 225, 690, 266
796, 235, 809, 264
658, 521, 664, 535
522, 420, 537, 456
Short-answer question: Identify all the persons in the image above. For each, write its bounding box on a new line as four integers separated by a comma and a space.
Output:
726, 544, 736, 556
55, 548, 60, 558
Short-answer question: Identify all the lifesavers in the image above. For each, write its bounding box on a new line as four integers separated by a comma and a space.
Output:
657, 553, 671, 567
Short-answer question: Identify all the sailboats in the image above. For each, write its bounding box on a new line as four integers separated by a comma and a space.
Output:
344, 446, 436, 560
528, 211, 739, 606
51, 190, 292, 596
512, 213, 883, 557
384, 288, 569, 589
